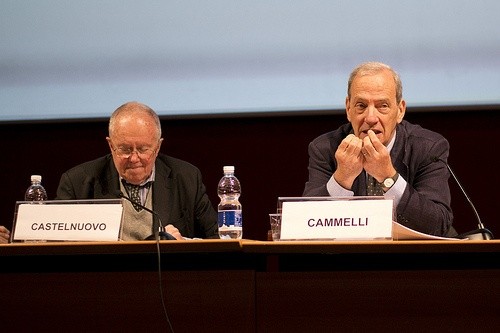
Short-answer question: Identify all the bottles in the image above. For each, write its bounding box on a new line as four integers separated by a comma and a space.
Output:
24, 174, 48, 242
218, 165, 243, 240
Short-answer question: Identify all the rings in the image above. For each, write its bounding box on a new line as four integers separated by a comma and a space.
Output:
363, 153, 367, 156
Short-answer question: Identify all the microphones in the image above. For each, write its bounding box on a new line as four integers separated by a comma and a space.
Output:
429, 155, 492, 240
114, 189, 177, 240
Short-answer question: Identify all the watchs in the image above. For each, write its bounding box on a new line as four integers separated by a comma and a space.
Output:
378, 172, 399, 192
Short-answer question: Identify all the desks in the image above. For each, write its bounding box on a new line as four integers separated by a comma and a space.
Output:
0, 239, 500, 333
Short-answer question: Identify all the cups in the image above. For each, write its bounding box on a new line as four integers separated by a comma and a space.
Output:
269, 213, 281, 241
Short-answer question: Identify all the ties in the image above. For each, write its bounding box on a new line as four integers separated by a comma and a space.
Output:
124, 181, 150, 211
367, 172, 384, 196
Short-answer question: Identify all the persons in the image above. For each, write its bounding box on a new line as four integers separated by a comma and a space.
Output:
0, 101, 222, 244
302, 63, 458, 238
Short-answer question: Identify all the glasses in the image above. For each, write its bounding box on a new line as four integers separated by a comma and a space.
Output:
109, 140, 161, 159
